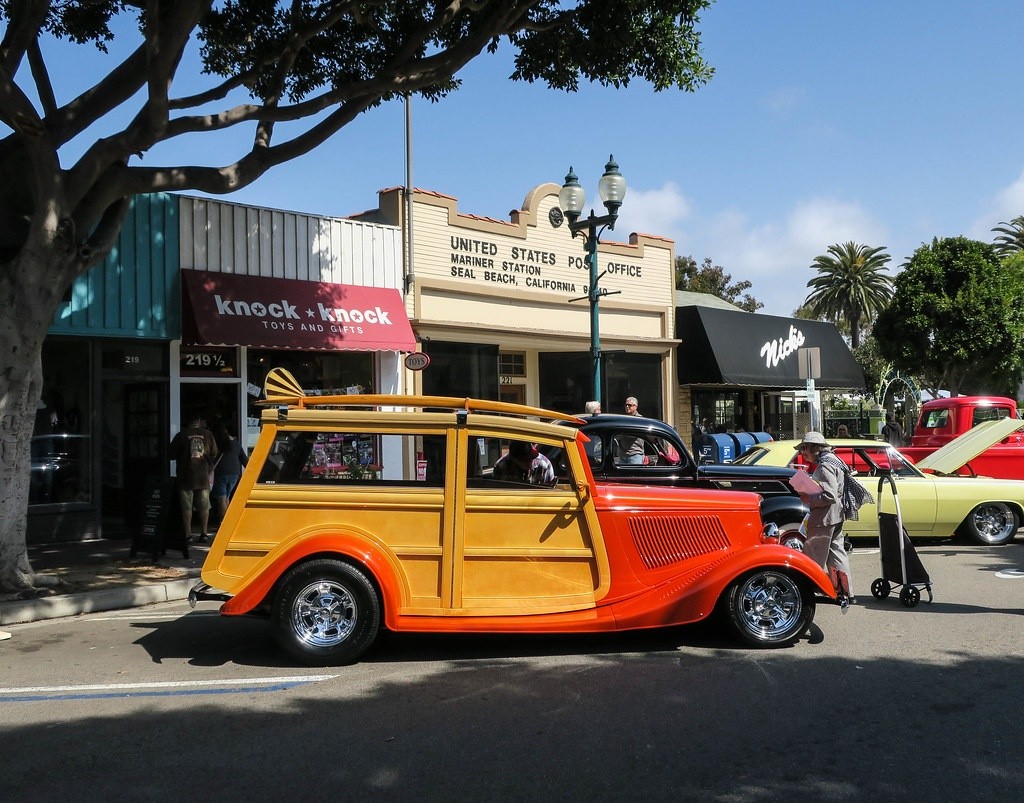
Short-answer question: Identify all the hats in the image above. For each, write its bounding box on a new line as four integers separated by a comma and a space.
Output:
792, 432, 832, 450
509, 440, 539, 462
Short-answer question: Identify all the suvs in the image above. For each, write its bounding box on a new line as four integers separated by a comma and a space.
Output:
187, 394, 837, 666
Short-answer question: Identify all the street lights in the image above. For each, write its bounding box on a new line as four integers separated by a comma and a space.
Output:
559, 154, 626, 404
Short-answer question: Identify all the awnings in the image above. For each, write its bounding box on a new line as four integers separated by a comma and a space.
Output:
675, 305, 866, 390
181, 268, 416, 354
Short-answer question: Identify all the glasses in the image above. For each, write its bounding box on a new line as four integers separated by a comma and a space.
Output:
801, 446, 807, 451
624, 403, 636, 407
839, 429, 845, 431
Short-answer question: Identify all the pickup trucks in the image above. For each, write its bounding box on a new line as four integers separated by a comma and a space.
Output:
795, 396, 1024, 480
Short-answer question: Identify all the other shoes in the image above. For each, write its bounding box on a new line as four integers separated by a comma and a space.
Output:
186, 536, 193, 544
835, 596, 857, 605
198, 533, 209, 543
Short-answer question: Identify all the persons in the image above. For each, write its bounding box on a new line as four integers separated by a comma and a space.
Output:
492, 440, 554, 485
616, 397, 645, 464
882, 412, 904, 447
794, 432, 857, 604
836, 425, 851, 438
213, 423, 248, 518
170, 415, 218, 543
583, 401, 602, 464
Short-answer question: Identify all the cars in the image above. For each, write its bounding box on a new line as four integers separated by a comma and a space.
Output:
731, 439, 1024, 546
483, 414, 853, 556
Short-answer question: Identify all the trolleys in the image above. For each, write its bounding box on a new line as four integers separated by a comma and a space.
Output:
871, 475, 933, 607
